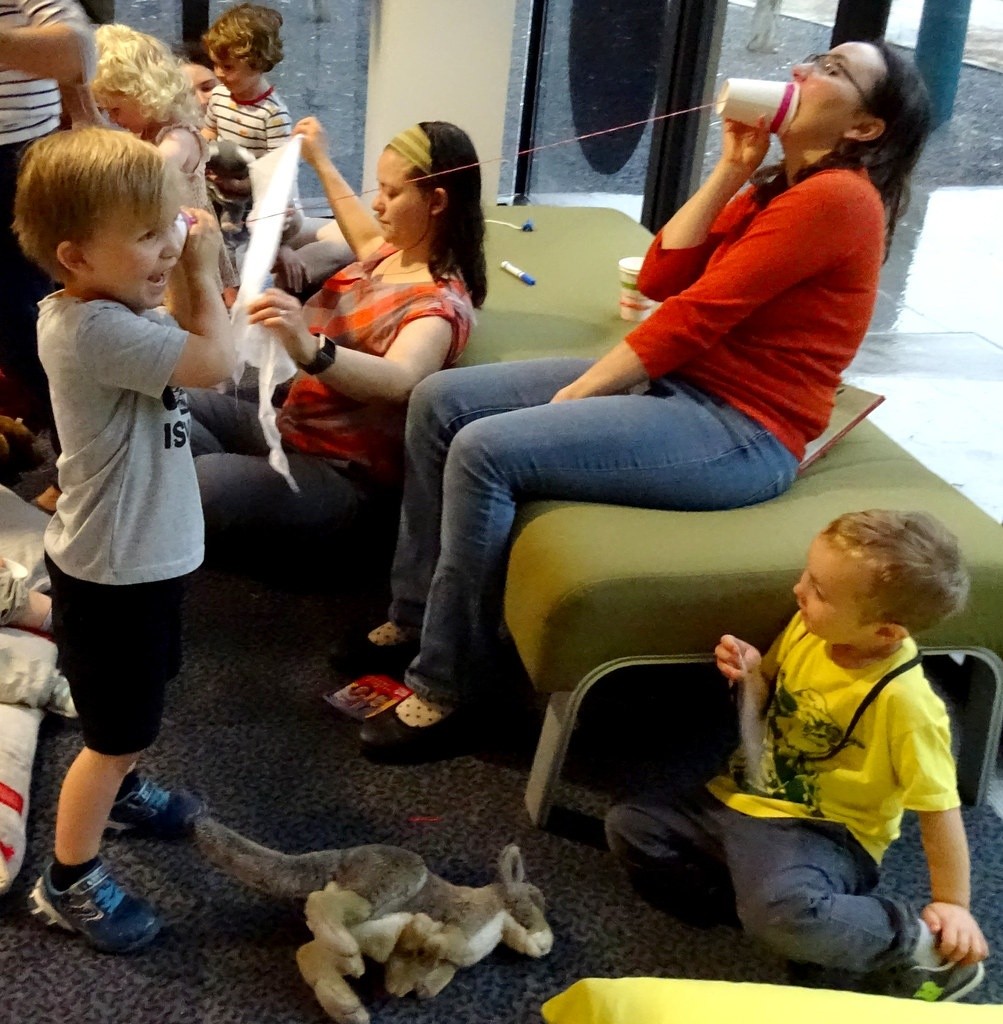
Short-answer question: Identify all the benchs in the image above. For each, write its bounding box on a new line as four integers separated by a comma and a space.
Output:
440, 205, 1003, 831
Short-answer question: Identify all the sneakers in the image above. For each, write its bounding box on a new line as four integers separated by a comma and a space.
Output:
828, 958, 986, 1004
101, 768, 203, 841
26, 861, 160, 953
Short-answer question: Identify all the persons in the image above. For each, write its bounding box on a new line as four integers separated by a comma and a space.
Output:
325, 39, 931, 766
204, 4, 305, 240
188, 122, 483, 529
0, 0, 311, 716
608, 509, 990, 1005
14, 129, 237, 953
94, 24, 234, 297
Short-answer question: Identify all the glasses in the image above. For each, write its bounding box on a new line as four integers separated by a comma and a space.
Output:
801, 52, 868, 108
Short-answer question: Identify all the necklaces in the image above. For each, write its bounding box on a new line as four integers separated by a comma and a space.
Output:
371, 255, 428, 281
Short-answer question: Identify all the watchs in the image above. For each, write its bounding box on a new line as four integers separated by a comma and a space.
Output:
298, 334, 335, 375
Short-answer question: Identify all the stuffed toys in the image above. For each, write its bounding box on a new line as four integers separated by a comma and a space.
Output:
193, 817, 555, 1024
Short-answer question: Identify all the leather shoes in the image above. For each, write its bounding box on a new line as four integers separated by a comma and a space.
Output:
357, 692, 456, 754
327, 630, 419, 676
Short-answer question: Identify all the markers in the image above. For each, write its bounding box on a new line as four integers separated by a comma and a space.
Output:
500, 259, 536, 287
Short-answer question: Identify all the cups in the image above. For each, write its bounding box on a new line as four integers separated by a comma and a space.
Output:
618, 256, 653, 322
716, 78, 800, 138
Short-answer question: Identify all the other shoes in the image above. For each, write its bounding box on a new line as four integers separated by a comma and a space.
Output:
29, 481, 61, 516
